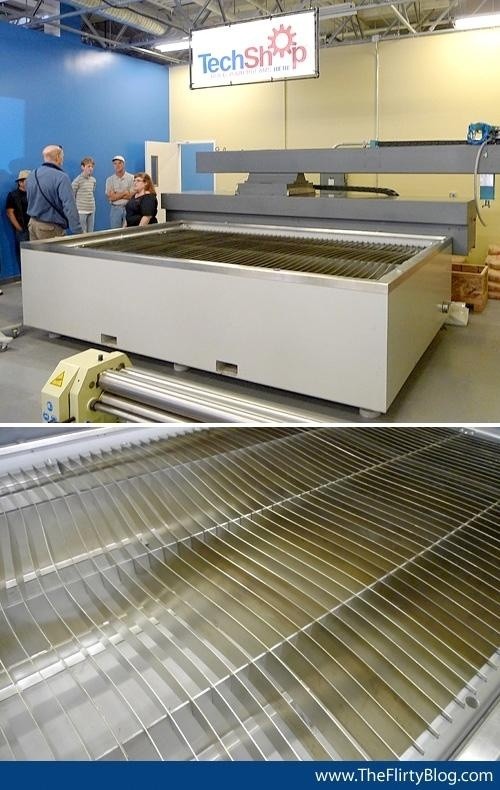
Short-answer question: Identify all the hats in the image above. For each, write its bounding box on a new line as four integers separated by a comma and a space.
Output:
112, 155, 125, 163
15, 169, 31, 182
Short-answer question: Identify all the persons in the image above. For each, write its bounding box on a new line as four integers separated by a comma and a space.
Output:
72, 157, 97, 233
105, 155, 135, 228
24, 145, 84, 239
6, 170, 31, 277
123, 172, 158, 228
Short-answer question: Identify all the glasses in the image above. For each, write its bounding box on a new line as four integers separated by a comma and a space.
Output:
56, 144, 63, 155
